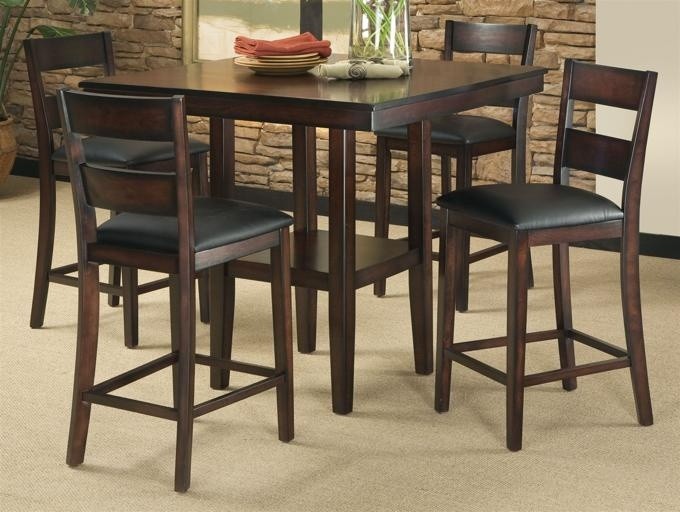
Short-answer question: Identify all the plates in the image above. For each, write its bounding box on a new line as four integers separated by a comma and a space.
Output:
230, 52, 326, 74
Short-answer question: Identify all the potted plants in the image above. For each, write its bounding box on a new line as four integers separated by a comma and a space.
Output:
0, 0, 98, 188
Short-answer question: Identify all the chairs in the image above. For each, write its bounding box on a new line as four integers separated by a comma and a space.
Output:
433, 58, 658, 455
23, 32, 212, 349
51, 85, 297, 490
372, 20, 539, 303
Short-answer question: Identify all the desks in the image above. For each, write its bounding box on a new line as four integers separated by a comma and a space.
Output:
80, 52, 548, 418
